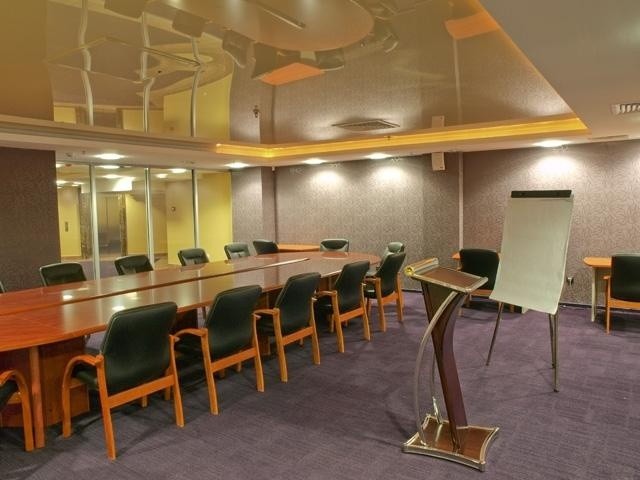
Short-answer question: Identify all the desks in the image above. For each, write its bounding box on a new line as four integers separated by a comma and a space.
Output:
450, 250, 515, 315
257, 57, 324, 88
273, 243, 321, 251
0, 250, 381, 427
444, 11, 500, 42
580, 255, 611, 324
153, 0, 373, 51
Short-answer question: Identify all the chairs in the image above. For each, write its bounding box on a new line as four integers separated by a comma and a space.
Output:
253, 239, 277, 255
38, 262, 86, 286
343, 245, 404, 333
320, 239, 349, 252
114, 254, 154, 276
316, 49, 345, 71
170, 10, 207, 37
234, 272, 322, 383
222, 30, 252, 68
160, 285, 265, 415
177, 248, 209, 266
249, 43, 277, 79
225, 242, 250, 259
0, 352, 35, 451
455, 249, 506, 313
366, 241, 404, 282
370, 21, 402, 52
103, 1, 147, 20
604, 252, 640, 334
62, 300, 187, 461
298, 260, 372, 354
